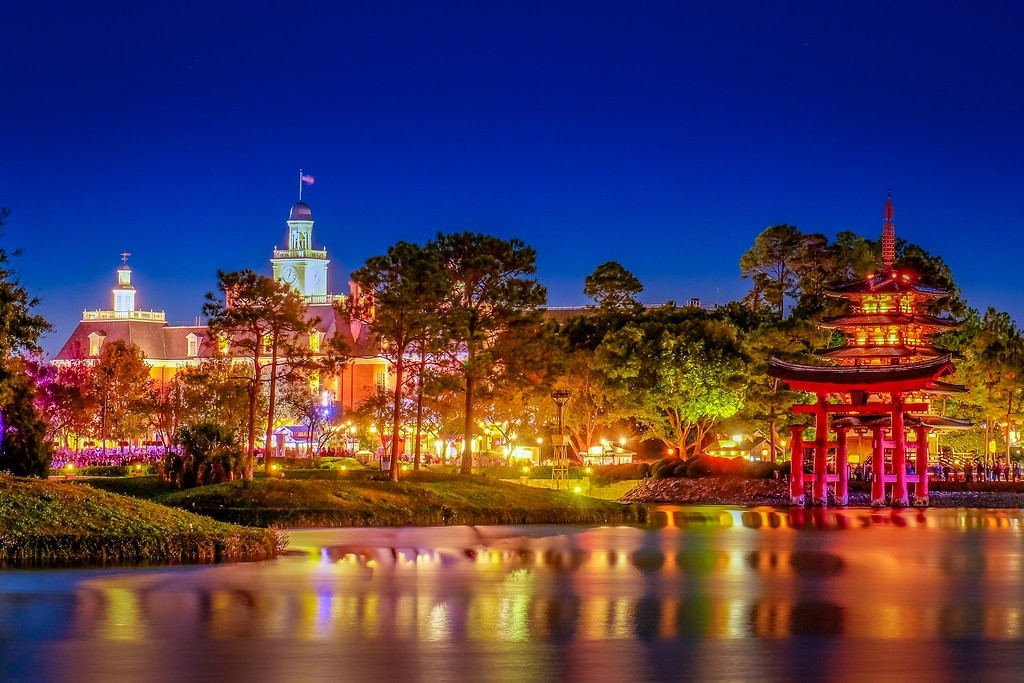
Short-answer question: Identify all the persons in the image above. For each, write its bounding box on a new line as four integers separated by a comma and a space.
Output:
49, 442, 613, 472
802, 457, 1021, 484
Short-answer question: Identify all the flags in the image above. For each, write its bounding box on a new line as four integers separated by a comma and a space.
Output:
301, 174, 314, 184
